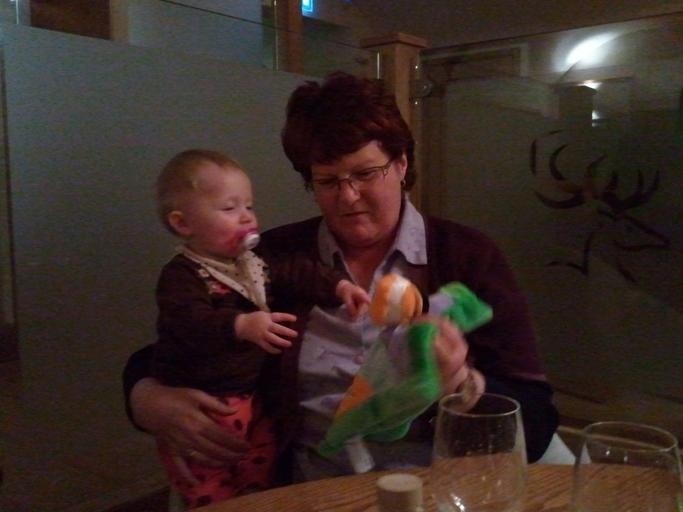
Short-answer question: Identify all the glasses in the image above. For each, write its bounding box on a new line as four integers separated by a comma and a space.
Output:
303, 157, 394, 192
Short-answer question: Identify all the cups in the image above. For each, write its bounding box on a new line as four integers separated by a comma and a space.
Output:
429, 390, 531, 511
569, 420, 682, 512
